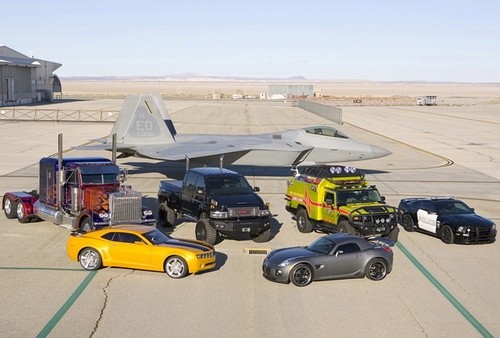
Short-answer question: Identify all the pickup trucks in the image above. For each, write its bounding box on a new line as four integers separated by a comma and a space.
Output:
157, 151, 271, 245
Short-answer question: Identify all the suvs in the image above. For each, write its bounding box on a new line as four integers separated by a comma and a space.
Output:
1, 129, 155, 235
284, 164, 399, 237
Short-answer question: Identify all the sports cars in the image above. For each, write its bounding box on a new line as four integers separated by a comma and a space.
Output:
68, 222, 217, 279
398, 196, 497, 245
262, 233, 394, 287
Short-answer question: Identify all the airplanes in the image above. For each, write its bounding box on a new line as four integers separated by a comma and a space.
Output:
72, 92, 393, 170
